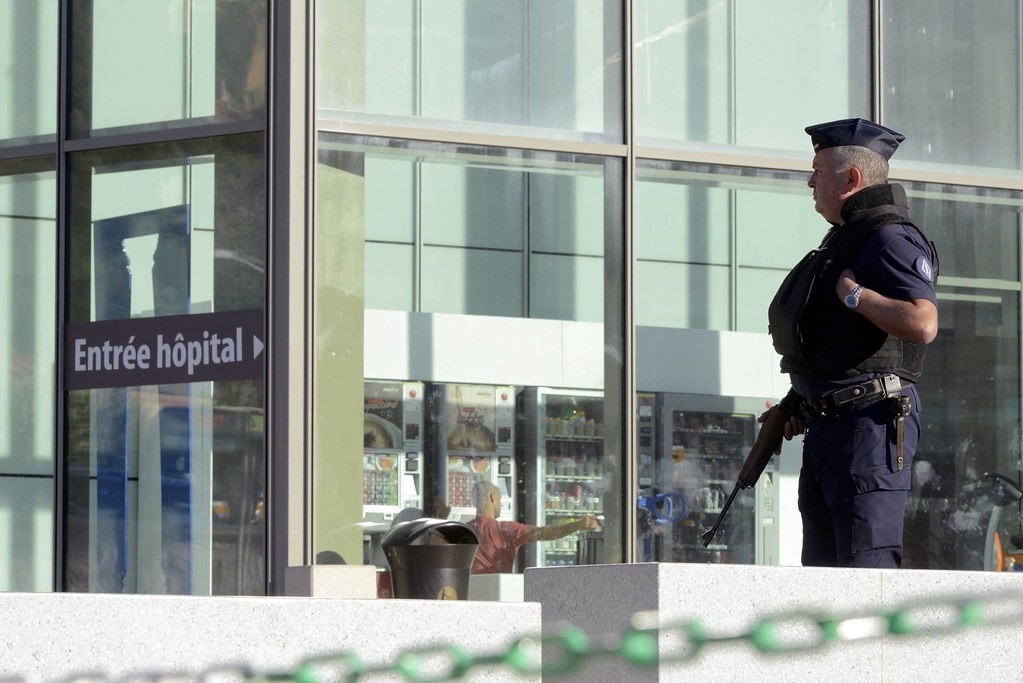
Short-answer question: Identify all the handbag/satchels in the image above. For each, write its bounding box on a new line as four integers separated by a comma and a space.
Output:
768, 246, 838, 361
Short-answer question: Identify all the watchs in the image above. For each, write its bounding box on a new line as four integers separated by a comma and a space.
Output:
844, 284, 864, 310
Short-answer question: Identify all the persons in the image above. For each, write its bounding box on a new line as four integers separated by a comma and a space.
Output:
462, 481, 601, 575
757, 118, 941, 569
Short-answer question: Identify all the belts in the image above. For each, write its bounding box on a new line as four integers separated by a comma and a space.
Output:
798, 371, 914, 425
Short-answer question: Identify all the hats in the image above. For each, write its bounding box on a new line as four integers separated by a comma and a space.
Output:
805, 118, 907, 160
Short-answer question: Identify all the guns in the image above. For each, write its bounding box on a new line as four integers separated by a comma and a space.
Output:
701, 388, 804, 548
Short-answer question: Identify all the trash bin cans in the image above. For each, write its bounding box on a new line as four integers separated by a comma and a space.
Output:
574, 530, 605, 565
380, 517, 479, 599
